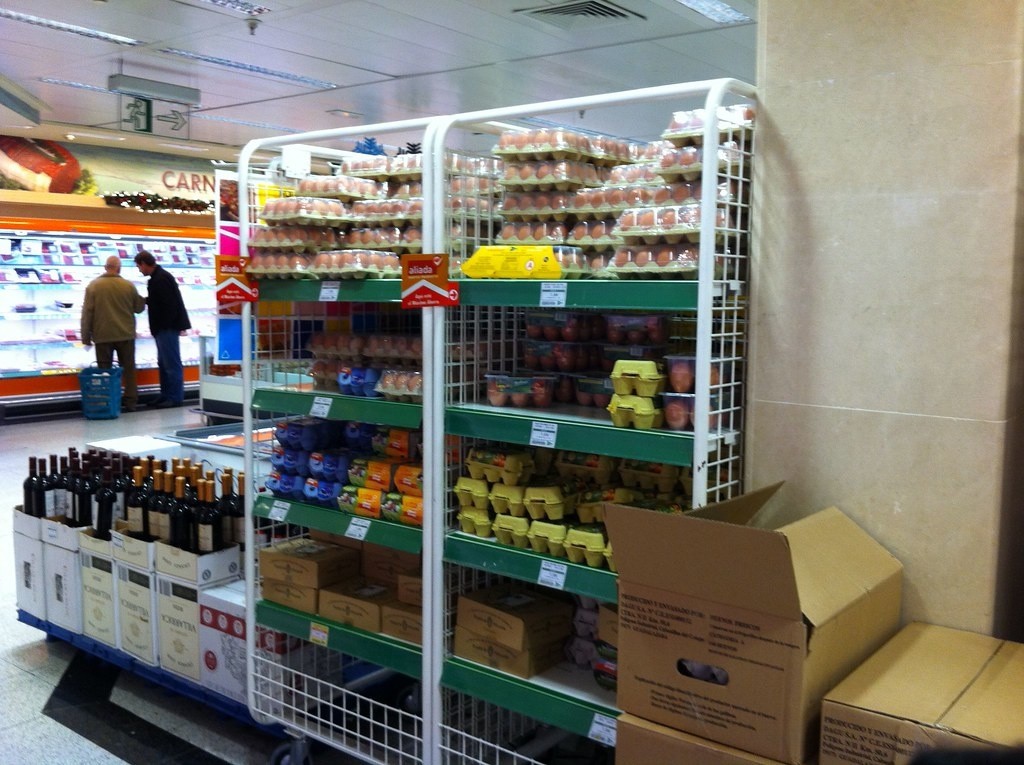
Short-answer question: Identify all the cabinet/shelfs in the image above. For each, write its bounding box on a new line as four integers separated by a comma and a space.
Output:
428, 77, 757, 765
236, 113, 535, 765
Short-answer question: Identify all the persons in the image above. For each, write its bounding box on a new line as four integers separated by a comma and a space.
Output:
81, 255, 145, 412
133, 250, 189, 408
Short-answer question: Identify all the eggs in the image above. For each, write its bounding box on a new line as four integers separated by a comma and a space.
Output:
248, 107, 756, 430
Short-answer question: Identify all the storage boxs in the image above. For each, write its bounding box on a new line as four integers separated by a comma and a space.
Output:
254, 526, 429, 647
13, 502, 342, 712
594, 478, 1024, 765
446, 584, 577, 679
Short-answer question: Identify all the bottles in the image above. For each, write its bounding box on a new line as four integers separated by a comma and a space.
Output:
23, 447, 301, 562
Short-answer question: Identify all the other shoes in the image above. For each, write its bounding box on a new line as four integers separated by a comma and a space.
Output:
125, 402, 147, 412
150, 399, 182, 409
